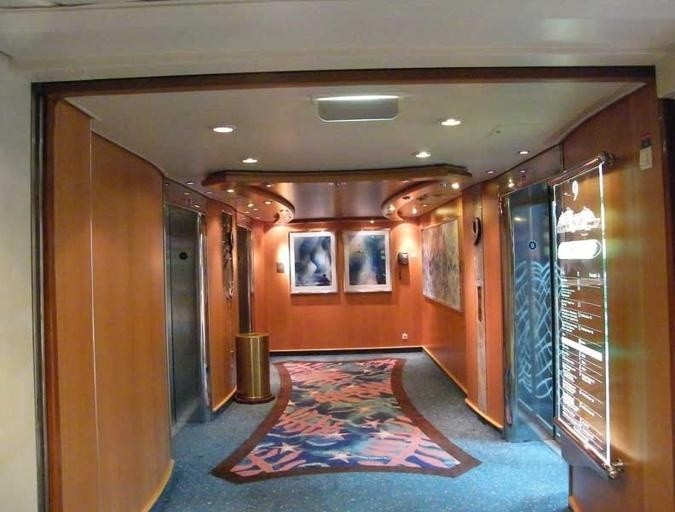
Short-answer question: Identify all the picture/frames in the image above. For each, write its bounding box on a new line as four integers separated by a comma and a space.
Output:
288, 230, 339, 296
420, 216, 463, 313
341, 228, 392, 293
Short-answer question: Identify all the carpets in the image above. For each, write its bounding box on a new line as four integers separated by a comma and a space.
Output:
207, 358, 483, 484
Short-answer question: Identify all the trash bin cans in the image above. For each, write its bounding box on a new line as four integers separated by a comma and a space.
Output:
234, 332, 274, 404
